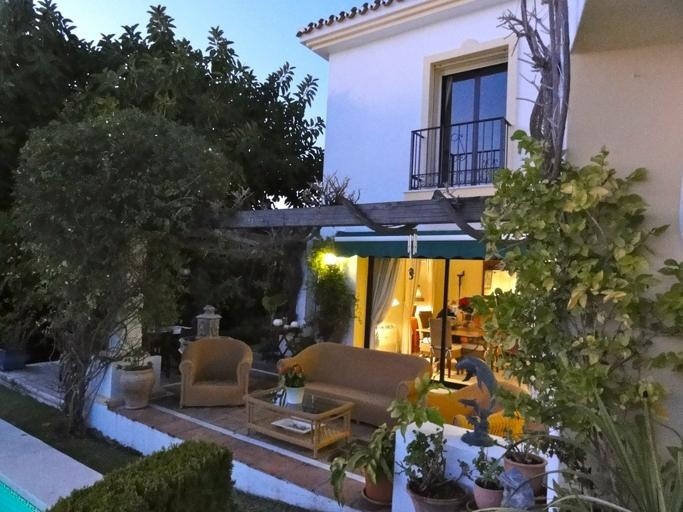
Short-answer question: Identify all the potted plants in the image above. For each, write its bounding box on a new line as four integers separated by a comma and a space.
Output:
118, 344, 155, 410
330, 371, 597, 511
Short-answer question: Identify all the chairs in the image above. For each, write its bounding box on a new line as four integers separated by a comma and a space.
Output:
410, 312, 511, 378
179, 335, 253, 410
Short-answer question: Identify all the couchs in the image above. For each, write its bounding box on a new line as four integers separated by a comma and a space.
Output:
277, 342, 433, 430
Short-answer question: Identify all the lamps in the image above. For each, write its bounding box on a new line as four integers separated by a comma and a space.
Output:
412, 286, 424, 317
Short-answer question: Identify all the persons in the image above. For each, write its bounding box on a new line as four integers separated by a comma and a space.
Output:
436, 299, 462, 345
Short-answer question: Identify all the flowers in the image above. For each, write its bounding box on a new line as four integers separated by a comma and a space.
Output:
278, 363, 305, 387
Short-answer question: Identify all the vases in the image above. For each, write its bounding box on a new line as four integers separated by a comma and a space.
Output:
285, 385, 306, 405
